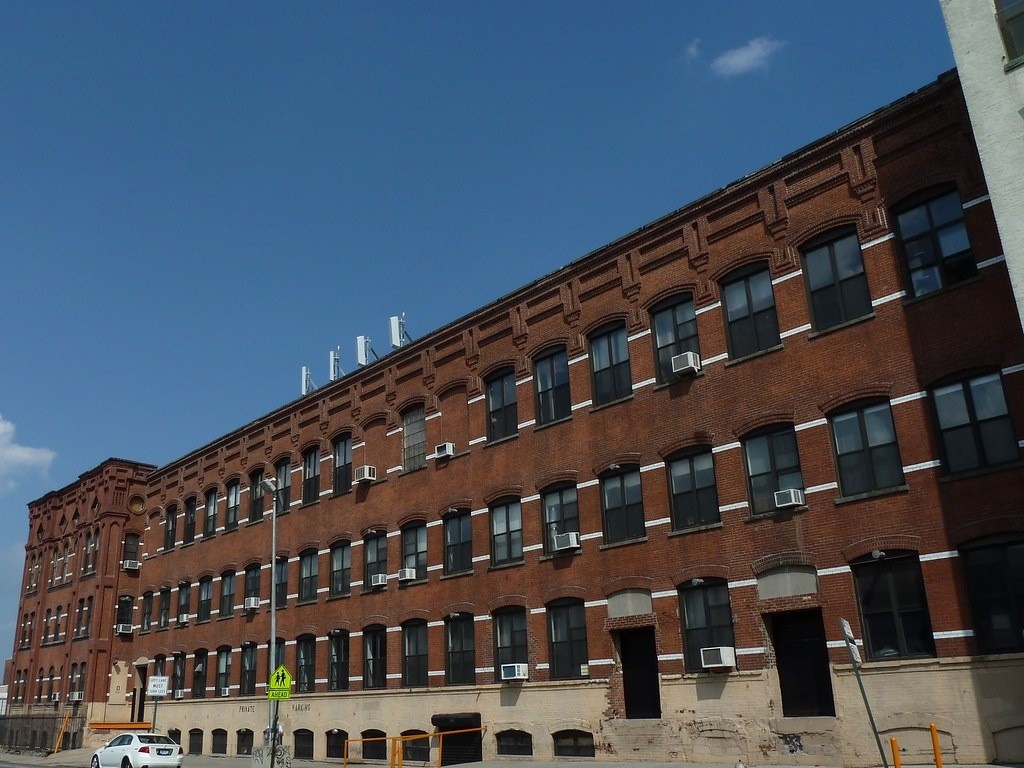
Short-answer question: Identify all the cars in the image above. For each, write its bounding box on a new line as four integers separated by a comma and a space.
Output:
90, 732, 184, 768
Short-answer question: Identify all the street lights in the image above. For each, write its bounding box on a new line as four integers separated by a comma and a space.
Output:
258, 478, 277, 768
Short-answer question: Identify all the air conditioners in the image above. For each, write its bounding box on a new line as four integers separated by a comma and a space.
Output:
554, 532, 580, 550
117, 624, 133, 633
52, 692, 60, 701
700, 647, 736, 668
221, 688, 230, 696
671, 351, 701, 374
775, 488, 805, 507
245, 597, 260, 608
372, 574, 388, 585
398, 569, 416, 580
123, 560, 139, 570
69, 692, 84, 701
355, 465, 376, 482
435, 443, 455, 459
501, 664, 529, 680
175, 690, 184, 698
